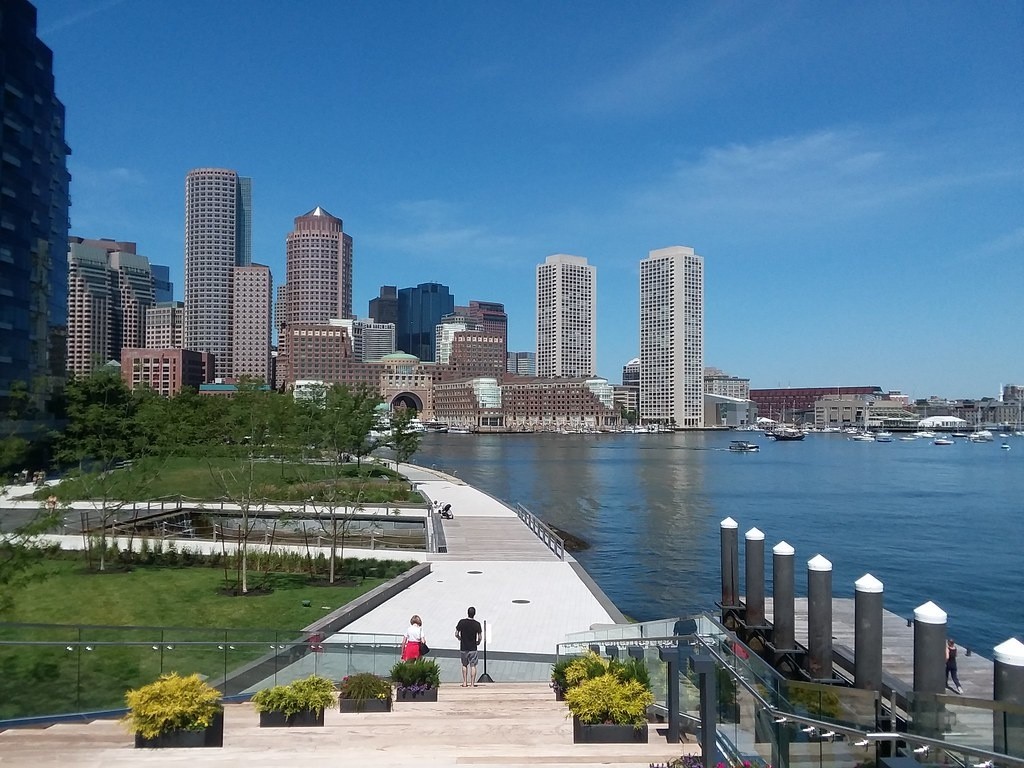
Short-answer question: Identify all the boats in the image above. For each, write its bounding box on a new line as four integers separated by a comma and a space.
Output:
1001, 444, 1009, 449
847, 430, 954, 444
559, 427, 675, 434
369, 415, 472, 437
729, 440, 760, 452
999, 433, 1012, 437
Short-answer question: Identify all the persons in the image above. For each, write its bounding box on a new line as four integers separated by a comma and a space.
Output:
946, 640, 963, 694
673, 619, 697, 645
400, 615, 426, 662
455, 607, 482, 687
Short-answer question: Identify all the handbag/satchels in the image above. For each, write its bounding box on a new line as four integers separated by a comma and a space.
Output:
420, 643, 430, 655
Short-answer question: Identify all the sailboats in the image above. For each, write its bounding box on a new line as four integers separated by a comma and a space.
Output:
950, 409, 967, 437
1014, 389, 1024, 436
968, 405, 994, 443
911, 405, 936, 438
764, 400, 805, 440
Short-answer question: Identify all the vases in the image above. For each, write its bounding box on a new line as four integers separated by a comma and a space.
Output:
340, 696, 391, 712
397, 687, 438, 701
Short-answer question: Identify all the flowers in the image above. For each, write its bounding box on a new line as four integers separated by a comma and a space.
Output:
341, 674, 391, 698
389, 657, 439, 692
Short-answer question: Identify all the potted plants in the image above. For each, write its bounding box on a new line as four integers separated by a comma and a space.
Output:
122, 672, 224, 747
249, 673, 337, 726
550, 652, 649, 743
